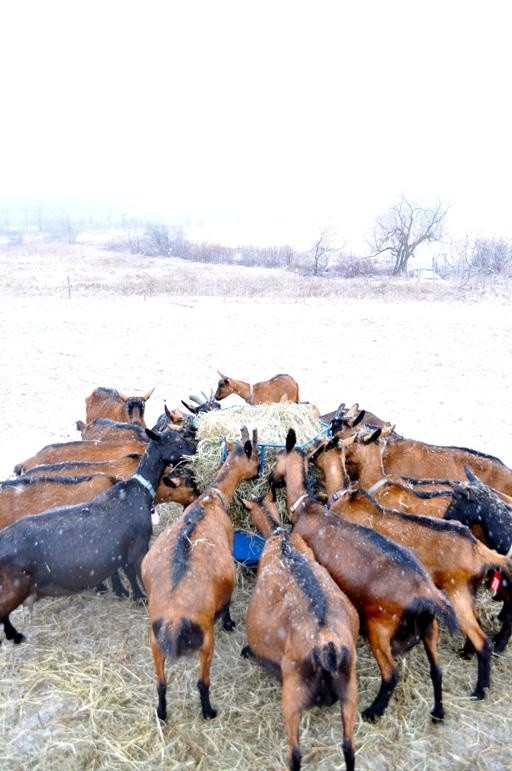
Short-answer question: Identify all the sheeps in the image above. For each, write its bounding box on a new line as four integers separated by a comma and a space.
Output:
0, 369, 512, 770
